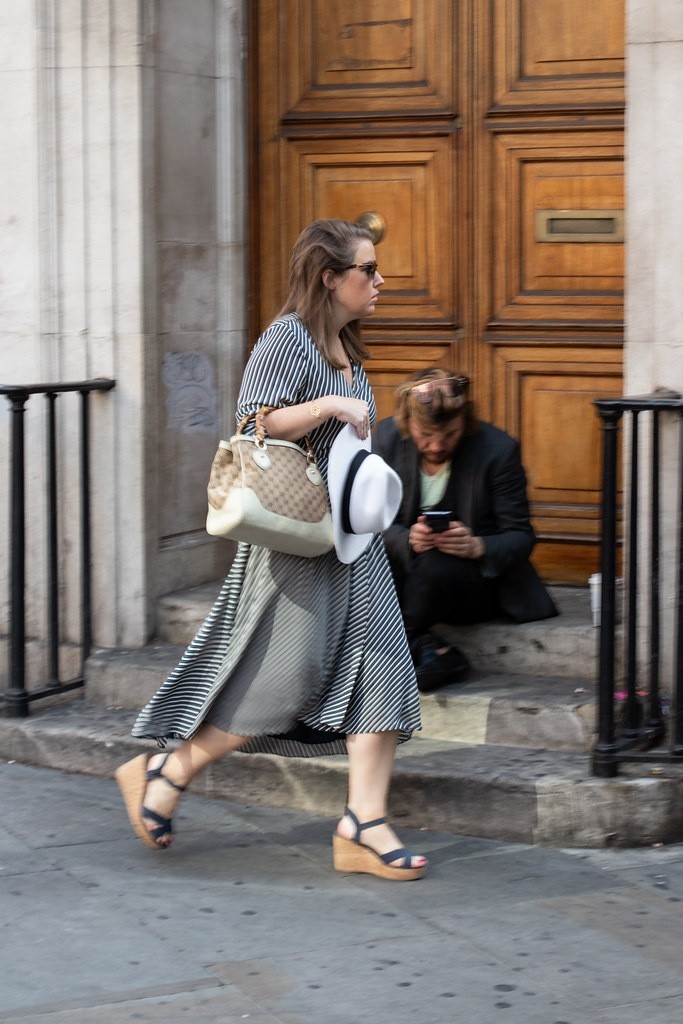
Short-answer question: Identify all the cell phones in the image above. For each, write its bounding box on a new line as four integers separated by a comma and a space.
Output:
424, 511, 450, 533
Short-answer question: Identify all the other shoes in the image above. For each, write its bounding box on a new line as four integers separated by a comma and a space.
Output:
403, 633, 424, 666
413, 646, 471, 692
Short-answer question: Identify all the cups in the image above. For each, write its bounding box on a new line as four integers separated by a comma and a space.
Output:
588, 573, 601, 626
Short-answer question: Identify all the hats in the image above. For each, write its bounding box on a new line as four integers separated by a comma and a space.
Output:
327, 422, 403, 564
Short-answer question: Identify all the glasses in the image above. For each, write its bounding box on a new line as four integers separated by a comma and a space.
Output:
318, 261, 377, 286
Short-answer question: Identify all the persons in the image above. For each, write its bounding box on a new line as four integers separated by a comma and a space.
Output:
369, 366, 560, 693
114, 218, 428, 882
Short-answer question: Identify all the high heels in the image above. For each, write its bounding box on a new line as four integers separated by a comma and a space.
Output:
115, 751, 187, 850
333, 807, 429, 880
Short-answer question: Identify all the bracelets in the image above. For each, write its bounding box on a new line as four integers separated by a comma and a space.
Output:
310, 400, 324, 424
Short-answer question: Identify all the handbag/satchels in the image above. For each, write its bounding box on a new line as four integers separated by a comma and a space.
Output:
206, 406, 334, 558
